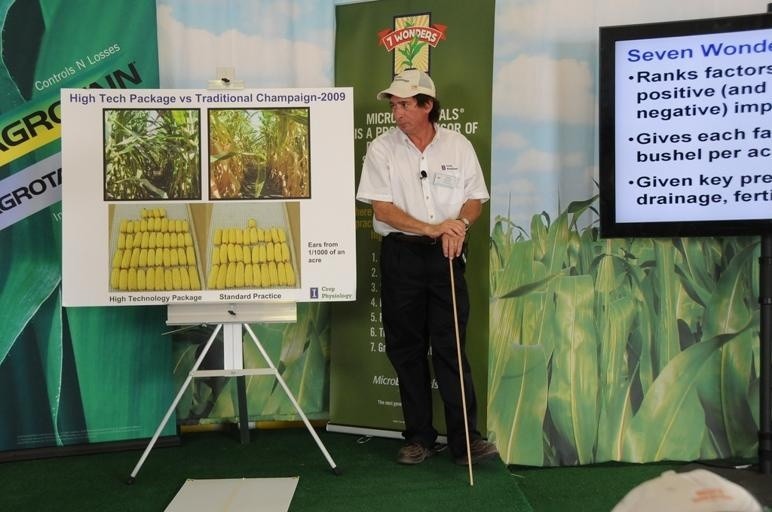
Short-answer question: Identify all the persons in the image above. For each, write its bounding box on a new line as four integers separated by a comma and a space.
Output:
356, 69, 499, 465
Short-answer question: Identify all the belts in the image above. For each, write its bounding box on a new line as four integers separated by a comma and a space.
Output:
382, 233, 440, 246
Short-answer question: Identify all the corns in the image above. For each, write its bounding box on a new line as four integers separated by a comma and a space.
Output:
110, 207, 202, 290
207, 217, 297, 290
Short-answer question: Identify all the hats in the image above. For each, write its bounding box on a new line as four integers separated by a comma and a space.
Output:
377, 69, 436, 101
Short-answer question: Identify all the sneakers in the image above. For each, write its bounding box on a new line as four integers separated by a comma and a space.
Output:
397, 441, 432, 465
456, 440, 497, 466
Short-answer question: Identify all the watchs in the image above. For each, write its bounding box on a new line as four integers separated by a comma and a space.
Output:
456, 216, 471, 233
